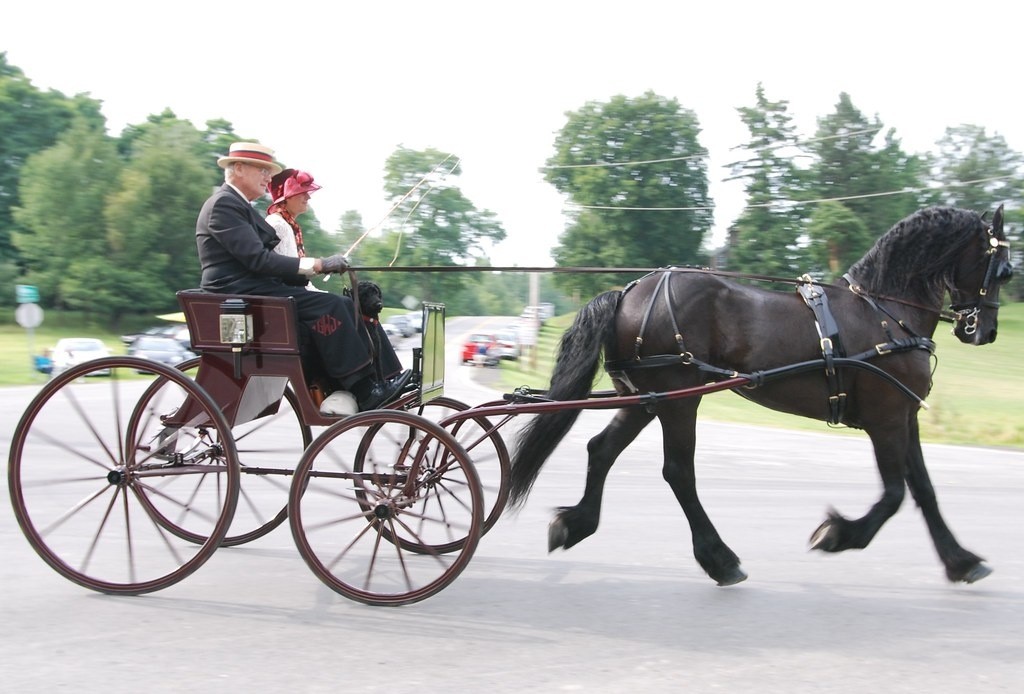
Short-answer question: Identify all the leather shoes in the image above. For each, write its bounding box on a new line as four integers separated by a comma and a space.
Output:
359, 369, 412, 411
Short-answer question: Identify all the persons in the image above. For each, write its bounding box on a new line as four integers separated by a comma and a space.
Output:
194, 142, 414, 411
264, 169, 331, 294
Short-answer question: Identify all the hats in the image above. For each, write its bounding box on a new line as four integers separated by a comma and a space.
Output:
267, 168, 323, 215
216, 142, 282, 177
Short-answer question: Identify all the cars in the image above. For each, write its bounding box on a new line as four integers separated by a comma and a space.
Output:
378, 310, 424, 349
49, 338, 114, 377
457, 301, 556, 367
119, 322, 199, 376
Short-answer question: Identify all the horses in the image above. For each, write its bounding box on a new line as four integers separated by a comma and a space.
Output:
502, 204, 1013, 586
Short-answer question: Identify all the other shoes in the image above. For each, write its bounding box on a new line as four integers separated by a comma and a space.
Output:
389, 370, 421, 392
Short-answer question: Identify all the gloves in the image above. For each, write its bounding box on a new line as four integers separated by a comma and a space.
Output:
319, 255, 349, 276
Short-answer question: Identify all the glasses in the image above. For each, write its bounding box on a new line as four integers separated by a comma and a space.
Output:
241, 162, 273, 178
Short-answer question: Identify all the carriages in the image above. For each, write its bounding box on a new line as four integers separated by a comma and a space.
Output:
6, 203, 1012, 608
